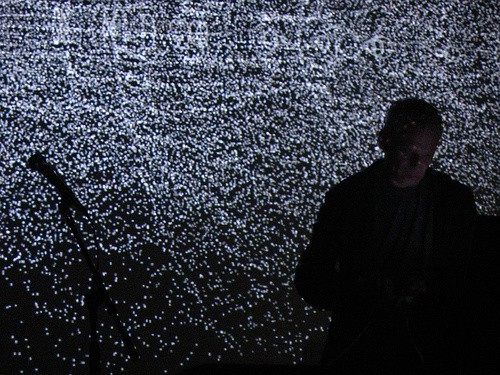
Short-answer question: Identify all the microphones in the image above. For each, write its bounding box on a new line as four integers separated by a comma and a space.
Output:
28, 154, 87, 215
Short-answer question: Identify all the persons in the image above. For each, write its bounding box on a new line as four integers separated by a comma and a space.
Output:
295, 97, 481, 375
